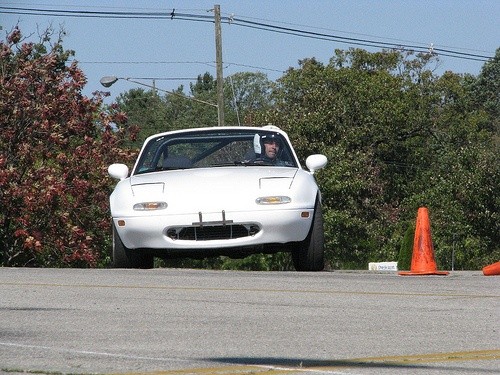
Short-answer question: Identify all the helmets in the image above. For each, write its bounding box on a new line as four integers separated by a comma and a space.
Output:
253, 125, 284, 156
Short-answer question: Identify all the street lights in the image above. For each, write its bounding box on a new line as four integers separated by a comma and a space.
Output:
99, 76, 218, 124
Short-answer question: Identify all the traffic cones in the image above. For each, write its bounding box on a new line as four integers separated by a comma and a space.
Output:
398, 207, 450, 276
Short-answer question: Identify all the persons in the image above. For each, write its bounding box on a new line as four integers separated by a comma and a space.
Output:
248, 137, 286, 166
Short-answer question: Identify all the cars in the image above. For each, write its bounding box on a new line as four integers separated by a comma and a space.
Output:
108, 124, 328, 271
